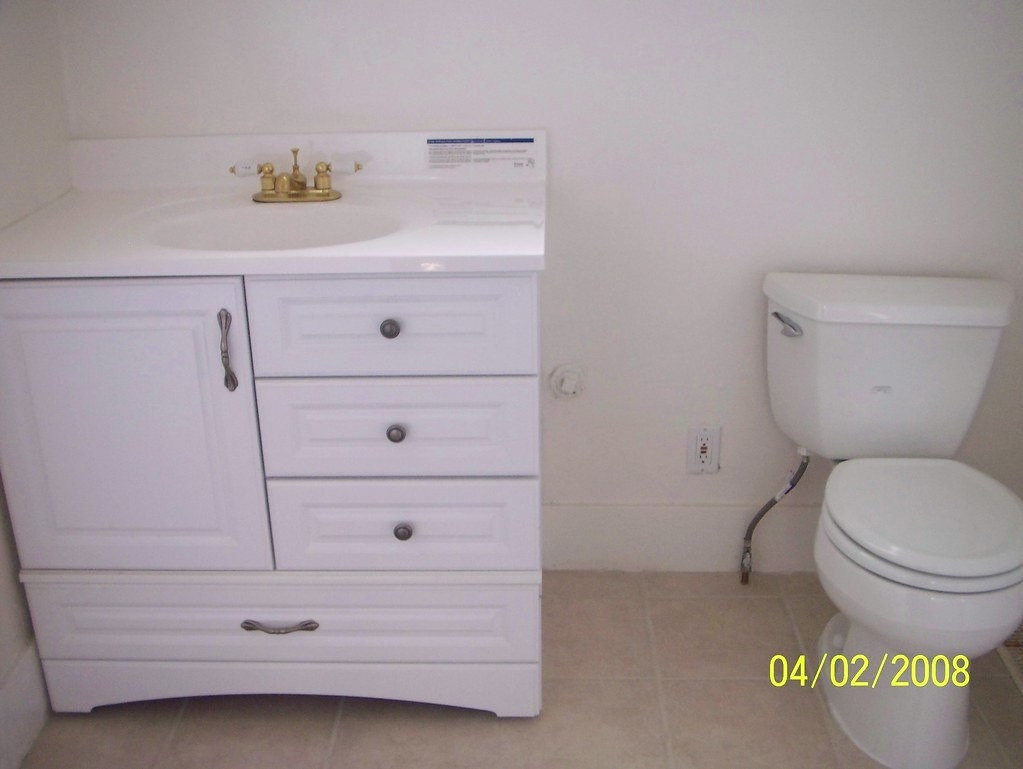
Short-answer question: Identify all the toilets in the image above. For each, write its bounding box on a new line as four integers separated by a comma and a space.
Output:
760, 270, 1023, 768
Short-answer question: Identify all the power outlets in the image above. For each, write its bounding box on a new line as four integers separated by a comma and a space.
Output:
687, 426, 721, 475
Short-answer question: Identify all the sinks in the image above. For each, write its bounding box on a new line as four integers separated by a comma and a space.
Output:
135, 199, 404, 252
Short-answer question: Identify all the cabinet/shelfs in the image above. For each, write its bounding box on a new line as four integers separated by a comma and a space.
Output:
0, 276, 542, 719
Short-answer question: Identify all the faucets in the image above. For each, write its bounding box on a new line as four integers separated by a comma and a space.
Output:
285, 145, 308, 197
